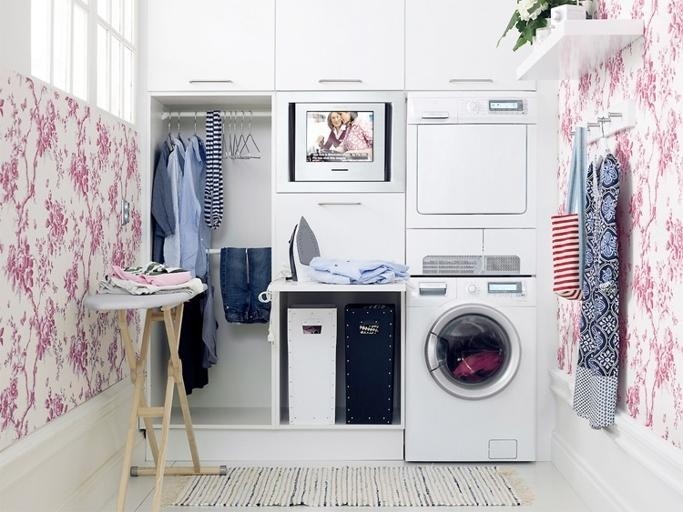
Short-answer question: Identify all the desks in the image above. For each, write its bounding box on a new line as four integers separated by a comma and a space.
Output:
82, 284, 226, 511
270, 281, 405, 428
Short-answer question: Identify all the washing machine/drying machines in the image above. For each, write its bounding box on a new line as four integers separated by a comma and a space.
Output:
407, 92, 539, 278
405, 276, 539, 464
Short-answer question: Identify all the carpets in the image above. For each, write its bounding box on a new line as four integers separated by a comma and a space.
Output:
173, 465, 523, 509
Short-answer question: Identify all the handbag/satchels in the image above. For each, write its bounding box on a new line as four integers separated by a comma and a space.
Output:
550, 213, 583, 301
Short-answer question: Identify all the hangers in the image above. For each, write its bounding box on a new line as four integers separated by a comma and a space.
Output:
167, 110, 263, 159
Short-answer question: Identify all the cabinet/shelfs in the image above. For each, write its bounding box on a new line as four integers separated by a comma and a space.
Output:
269, 0, 407, 281
404, 0, 537, 91
143, 0, 276, 93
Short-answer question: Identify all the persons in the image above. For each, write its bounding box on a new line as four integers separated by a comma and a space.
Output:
315, 112, 347, 151
327, 112, 372, 153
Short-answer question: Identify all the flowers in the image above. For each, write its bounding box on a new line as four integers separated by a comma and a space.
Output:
496, 0, 595, 52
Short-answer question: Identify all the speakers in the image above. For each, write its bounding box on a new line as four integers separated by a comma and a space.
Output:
385, 103, 391, 182
289, 103, 295, 182
